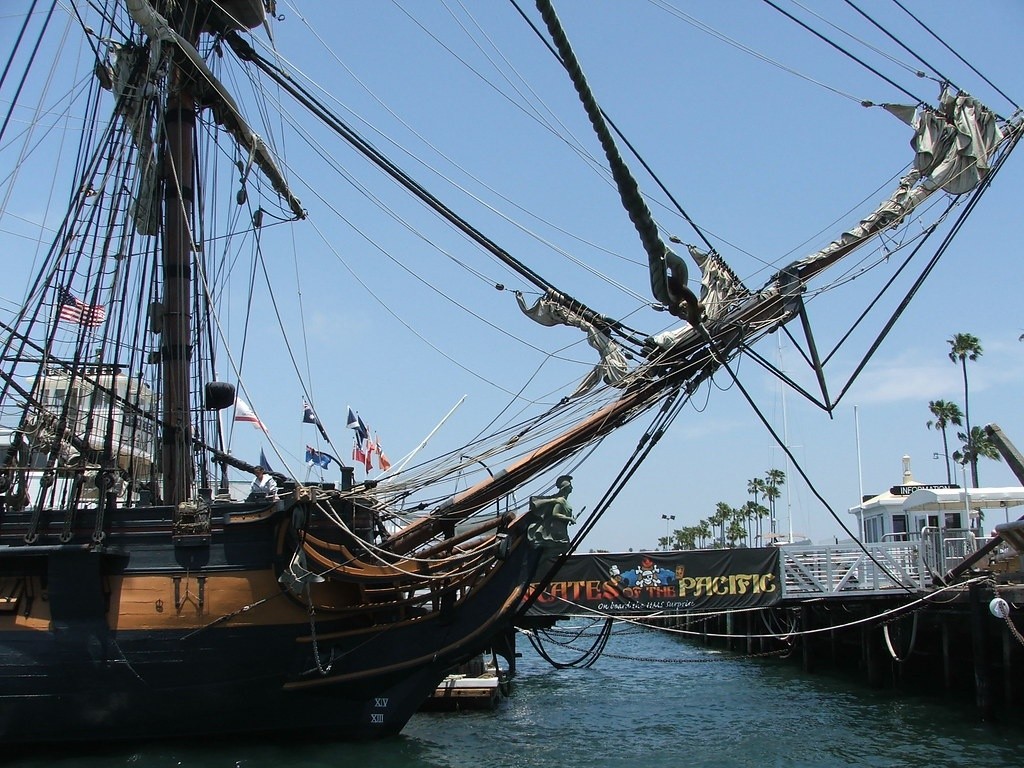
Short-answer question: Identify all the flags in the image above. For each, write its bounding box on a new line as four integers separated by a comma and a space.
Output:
260, 450, 272, 471
306, 447, 331, 470
376, 436, 390, 471
347, 408, 375, 474
302, 401, 329, 444
234, 397, 269, 433
57, 284, 105, 327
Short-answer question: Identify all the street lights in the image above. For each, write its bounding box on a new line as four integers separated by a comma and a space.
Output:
661, 514, 675, 550
933, 451, 957, 485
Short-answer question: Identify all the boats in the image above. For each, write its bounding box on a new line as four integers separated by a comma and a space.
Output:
1, 0, 1024, 754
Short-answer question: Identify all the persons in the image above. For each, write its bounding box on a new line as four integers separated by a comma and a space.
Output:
251, 466, 279, 502
550, 481, 576, 542
